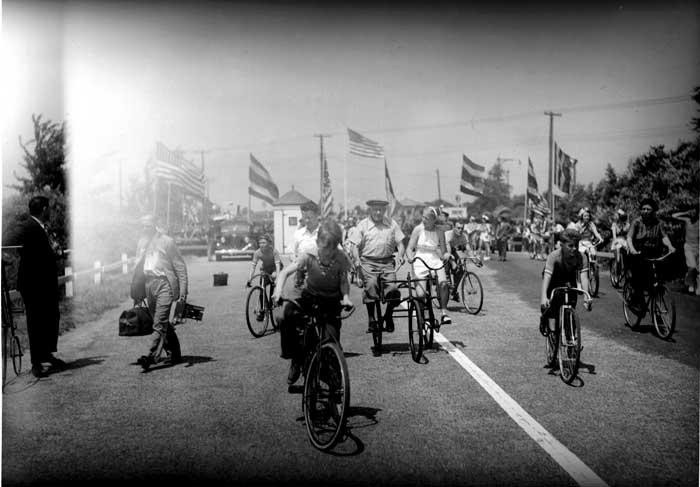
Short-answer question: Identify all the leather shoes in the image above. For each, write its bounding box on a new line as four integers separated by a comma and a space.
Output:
287, 360, 301, 384
384, 314, 394, 332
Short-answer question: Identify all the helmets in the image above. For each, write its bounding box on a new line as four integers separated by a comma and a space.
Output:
257, 235, 271, 242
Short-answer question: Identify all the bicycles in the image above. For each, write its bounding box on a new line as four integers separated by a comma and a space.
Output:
430, 255, 486, 314
3, 262, 24, 391
577, 240, 605, 298
543, 286, 594, 385
608, 242, 631, 286
274, 292, 359, 454
244, 272, 278, 338
525, 236, 552, 260
621, 247, 679, 340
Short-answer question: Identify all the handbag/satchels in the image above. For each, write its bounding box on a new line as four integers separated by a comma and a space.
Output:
119, 300, 154, 336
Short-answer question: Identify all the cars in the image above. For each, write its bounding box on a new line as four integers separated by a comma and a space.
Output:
213, 218, 258, 261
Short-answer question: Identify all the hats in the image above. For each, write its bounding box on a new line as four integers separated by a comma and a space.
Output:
366, 200, 389, 206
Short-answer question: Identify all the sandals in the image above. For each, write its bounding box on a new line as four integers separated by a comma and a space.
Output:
441, 315, 452, 325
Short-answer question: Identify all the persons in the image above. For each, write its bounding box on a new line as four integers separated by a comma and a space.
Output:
18, 196, 68, 376
627, 204, 676, 312
351, 199, 405, 332
406, 205, 455, 324
539, 229, 591, 356
274, 220, 353, 389
128, 215, 188, 369
672, 205, 699, 295
158, 206, 697, 279
246, 233, 281, 319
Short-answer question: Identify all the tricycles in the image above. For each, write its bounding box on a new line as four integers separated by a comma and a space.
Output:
351, 256, 462, 362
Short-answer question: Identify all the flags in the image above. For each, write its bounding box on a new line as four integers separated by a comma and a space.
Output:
460, 154, 485, 197
385, 165, 402, 207
527, 156, 542, 207
156, 140, 207, 197
553, 141, 578, 194
322, 158, 338, 220
346, 127, 385, 161
249, 155, 279, 205
